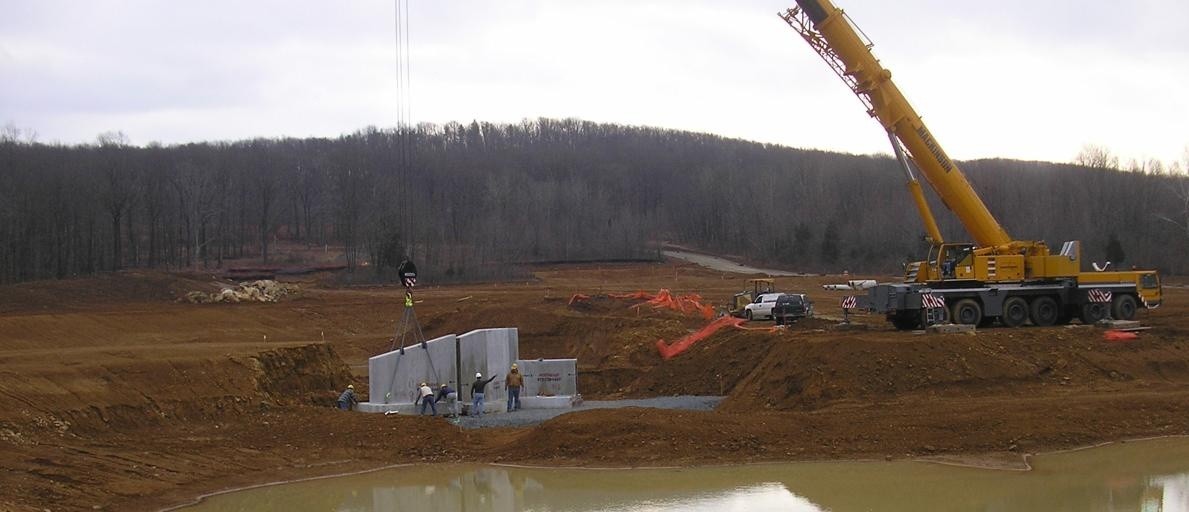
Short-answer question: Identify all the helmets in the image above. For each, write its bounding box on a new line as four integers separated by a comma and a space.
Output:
511, 364, 517, 369
476, 372, 481, 377
441, 383, 448, 389
347, 384, 355, 389
421, 382, 426, 386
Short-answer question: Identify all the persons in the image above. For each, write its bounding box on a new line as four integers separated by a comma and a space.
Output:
434, 383, 459, 417
415, 382, 438, 416
470, 372, 498, 417
504, 363, 524, 413
336, 385, 358, 410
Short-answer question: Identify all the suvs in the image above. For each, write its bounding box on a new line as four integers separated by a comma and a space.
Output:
745, 292, 786, 322
775, 293, 814, 325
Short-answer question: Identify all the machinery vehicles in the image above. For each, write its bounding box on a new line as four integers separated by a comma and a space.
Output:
778, 1, 1168, 332
727, 276, 775, 317
